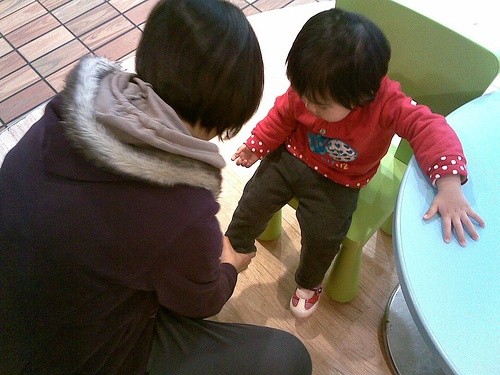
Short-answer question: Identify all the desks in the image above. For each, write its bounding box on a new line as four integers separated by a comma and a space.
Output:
383, 91, 500, 375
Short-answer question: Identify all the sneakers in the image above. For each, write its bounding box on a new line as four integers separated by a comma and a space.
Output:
289, 285, 324, 319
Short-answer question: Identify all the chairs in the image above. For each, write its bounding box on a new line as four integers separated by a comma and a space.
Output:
256, 0, 499, 304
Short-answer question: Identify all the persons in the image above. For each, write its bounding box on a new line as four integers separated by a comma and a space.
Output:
0, 0, 315, 375
225, 7, 486, 319
